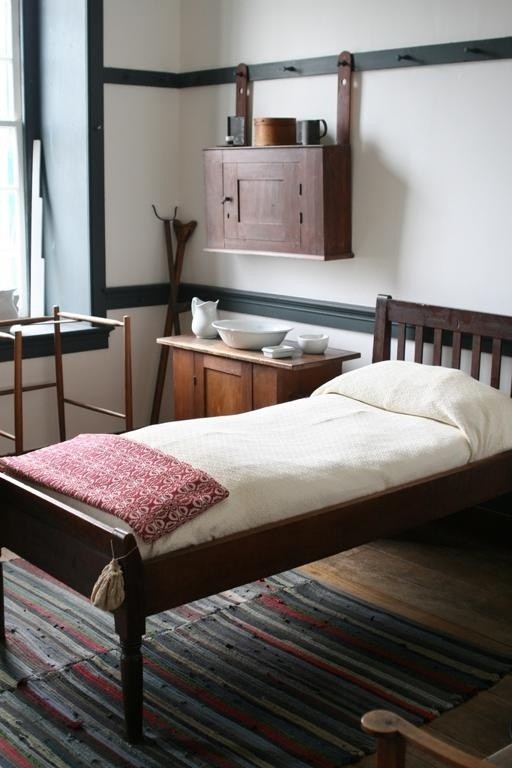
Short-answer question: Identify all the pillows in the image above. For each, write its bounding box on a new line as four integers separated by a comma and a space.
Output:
313, 358, 504, 447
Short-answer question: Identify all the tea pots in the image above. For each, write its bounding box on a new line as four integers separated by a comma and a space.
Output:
189, 295, 220, 338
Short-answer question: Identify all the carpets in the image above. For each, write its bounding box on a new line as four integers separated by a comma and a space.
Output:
0, 556, 508, 766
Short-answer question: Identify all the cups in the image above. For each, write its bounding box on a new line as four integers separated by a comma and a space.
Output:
299, 118, 327, 146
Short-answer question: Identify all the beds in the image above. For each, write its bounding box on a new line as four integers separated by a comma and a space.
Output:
0, 293, 510, 743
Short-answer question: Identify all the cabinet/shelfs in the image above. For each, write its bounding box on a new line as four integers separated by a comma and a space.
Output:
203, 143, 354, 262
155, 330, 361, 421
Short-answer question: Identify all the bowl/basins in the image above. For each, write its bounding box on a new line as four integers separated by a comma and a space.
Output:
212, 319, 294, 349
298, 332, 329, 354
